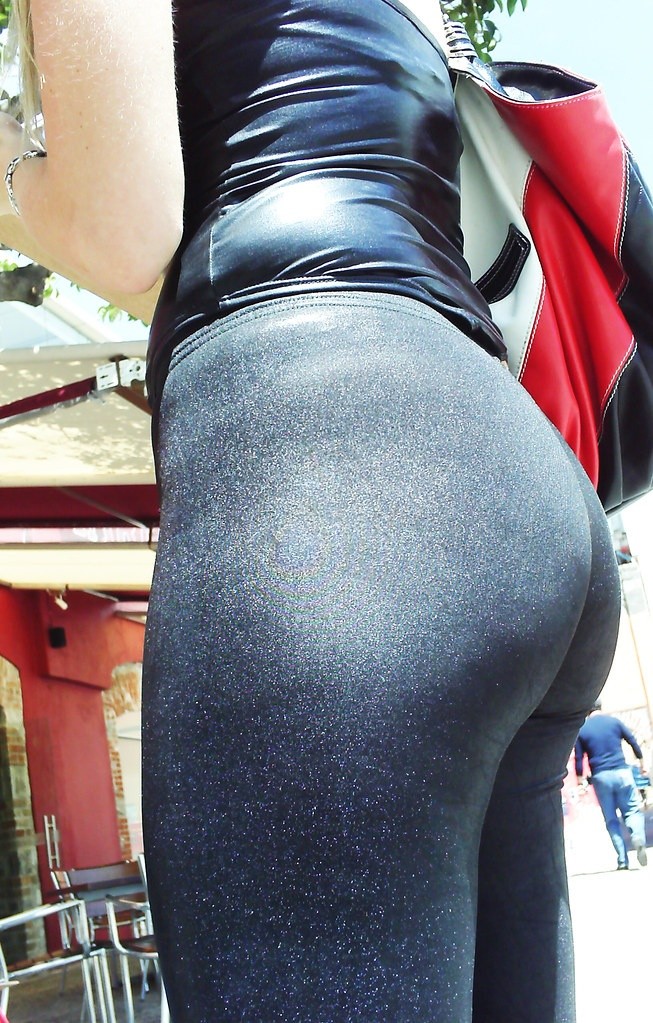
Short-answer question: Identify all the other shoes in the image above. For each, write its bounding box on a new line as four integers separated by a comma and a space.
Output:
616, 840, 646, 870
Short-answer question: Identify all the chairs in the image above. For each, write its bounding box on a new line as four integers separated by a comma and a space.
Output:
67, 856, 153, 1022
0, 899, 117, 1023
106, 855, 171, 1023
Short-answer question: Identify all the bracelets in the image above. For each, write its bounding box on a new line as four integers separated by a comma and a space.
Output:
3, 144, 54, 219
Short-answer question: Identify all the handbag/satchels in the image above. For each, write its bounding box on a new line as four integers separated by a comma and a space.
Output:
449, 21, 652, 514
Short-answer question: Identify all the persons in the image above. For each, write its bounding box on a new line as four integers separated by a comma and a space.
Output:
0, 0, 624, 1022
575, 695, 650, 870
